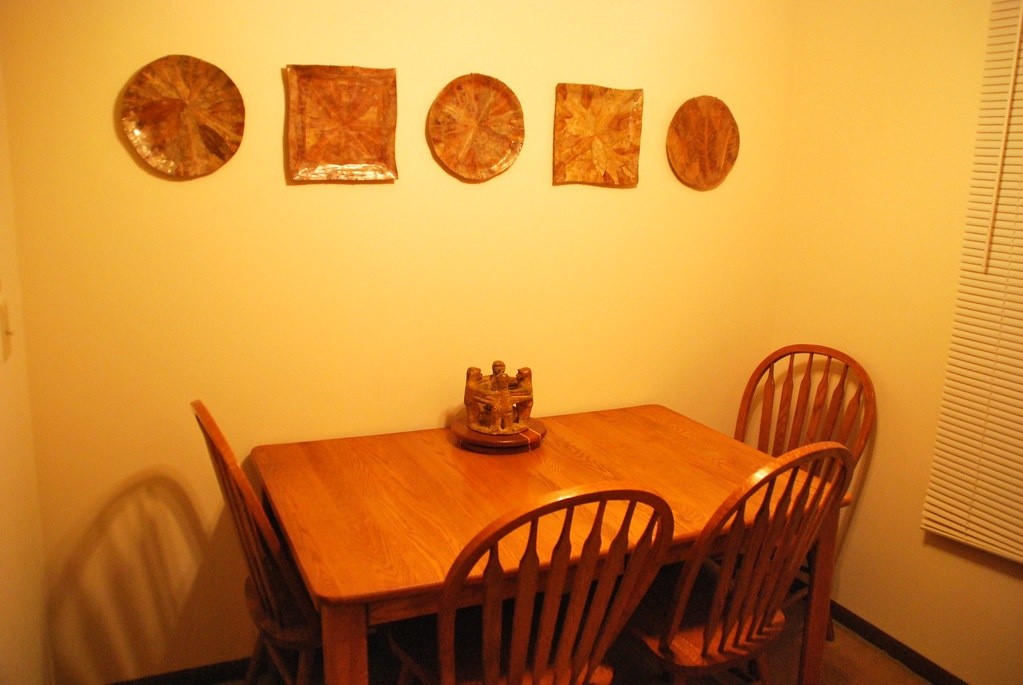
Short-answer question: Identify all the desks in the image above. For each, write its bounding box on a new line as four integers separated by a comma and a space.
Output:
249, 405, 838, 685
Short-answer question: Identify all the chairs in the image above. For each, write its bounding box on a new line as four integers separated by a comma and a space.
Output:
386, 480, 674, 684
703, 344, 878, 641
189, 397, 322, 684
627, 439, 853, 685
